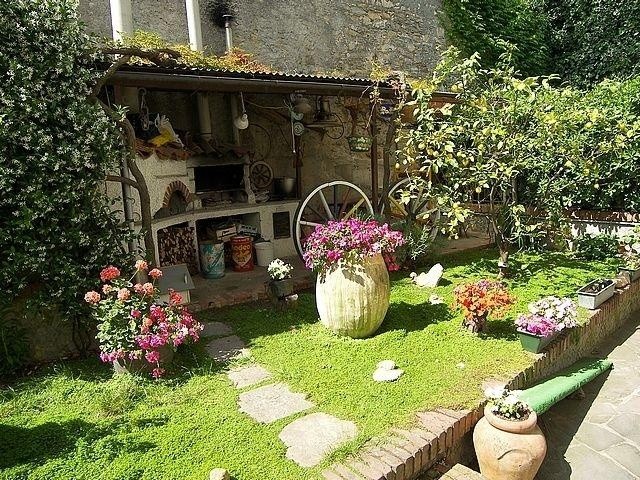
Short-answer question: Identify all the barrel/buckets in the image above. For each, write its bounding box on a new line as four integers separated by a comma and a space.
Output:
254, 241, 274, 266
198, 240, 225, 280
230, 236, 254, 272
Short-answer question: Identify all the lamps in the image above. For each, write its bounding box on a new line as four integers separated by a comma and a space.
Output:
233, 92, 250, 130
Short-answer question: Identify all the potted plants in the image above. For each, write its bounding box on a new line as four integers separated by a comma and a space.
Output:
618, 259, 640, 281
577, 277, 616, 310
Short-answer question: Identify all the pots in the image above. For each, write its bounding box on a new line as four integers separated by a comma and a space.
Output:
274, 174, 298, 194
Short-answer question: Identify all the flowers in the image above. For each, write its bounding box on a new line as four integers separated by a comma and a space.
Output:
485, 386, 531, 421
266, 257, 295, 280
514, 296, 582, 334
302, 216, 408, 280
83, 259, 206, 381
449, 279, 518, 328
